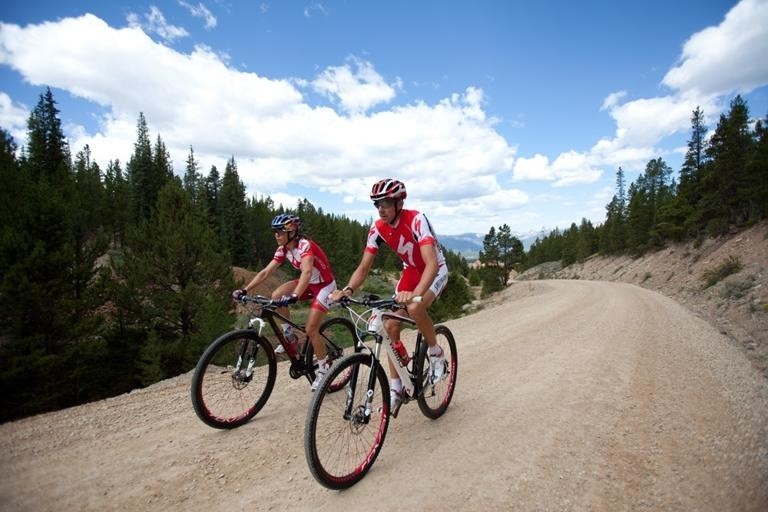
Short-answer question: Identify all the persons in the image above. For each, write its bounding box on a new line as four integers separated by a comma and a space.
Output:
330, 177, 449, 415
231, 213, 336, 392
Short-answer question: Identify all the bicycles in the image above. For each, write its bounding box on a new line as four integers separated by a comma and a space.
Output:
304, 292, 457, 489
191, 293, 361, 429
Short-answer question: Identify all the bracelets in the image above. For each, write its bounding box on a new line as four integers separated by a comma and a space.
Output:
342, 285, 353, 294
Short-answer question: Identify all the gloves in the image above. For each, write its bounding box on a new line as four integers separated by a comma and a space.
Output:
275, 292, 298, 307
231, 288, 247, 303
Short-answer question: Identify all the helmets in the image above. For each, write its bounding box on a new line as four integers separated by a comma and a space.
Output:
271, 214, 301, 232
370, 179, 406, 202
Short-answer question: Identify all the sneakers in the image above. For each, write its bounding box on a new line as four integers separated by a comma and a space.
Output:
428, 346, 444, 387
378, 385, 406, 415
310, 358, 333, 391
274, 334, 299, 354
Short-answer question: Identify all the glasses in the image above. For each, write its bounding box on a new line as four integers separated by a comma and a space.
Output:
372, 199, 397, 207
